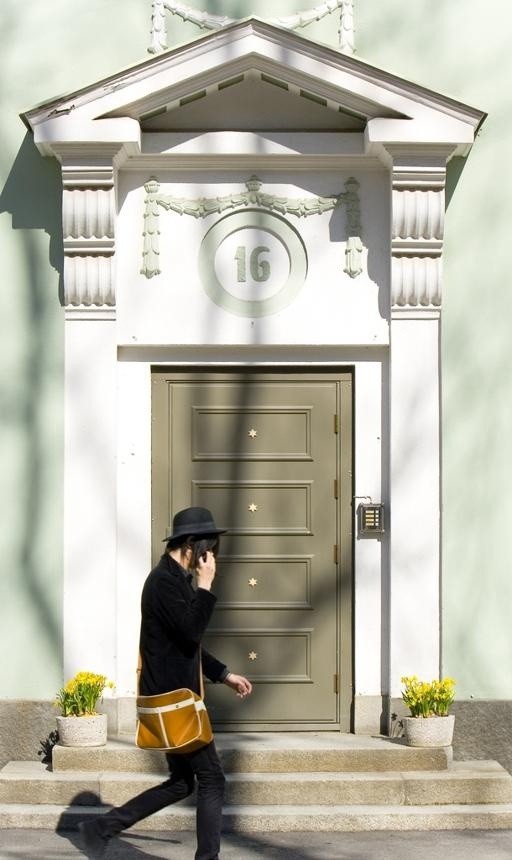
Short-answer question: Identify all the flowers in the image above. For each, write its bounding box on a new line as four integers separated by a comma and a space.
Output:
46, 671, 118, 713
397, 674, 456, 717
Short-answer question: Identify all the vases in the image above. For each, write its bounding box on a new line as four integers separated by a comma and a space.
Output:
56, 715, 108, 745
404, 714, 458, 746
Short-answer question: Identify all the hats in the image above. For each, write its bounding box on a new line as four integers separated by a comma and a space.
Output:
162, 507, 227, 541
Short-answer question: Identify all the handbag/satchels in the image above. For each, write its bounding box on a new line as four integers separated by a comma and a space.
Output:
135, 687, 214, 754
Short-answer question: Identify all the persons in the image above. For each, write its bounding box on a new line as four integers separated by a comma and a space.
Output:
74, 505, 254, 860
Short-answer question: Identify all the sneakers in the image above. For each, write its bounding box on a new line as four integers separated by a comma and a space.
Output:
77, 819, 108, 860
